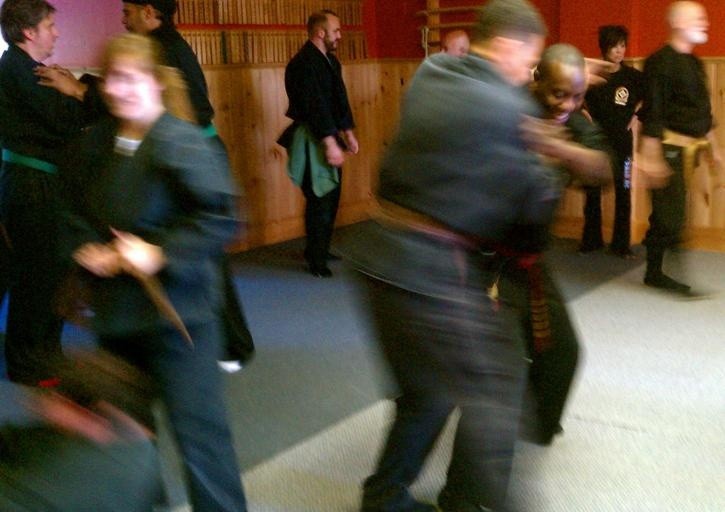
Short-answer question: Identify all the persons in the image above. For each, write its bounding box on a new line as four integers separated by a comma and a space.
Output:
57, 34, 248, 511
279, 12, 358, 276
350, 0, 572, 512
36, 1, 256, 374
638, 0, 723, 296
438, 28, 471, 61
580, 26, 650, 257
0, 2, 84, 384
485, 43, 617, 445
